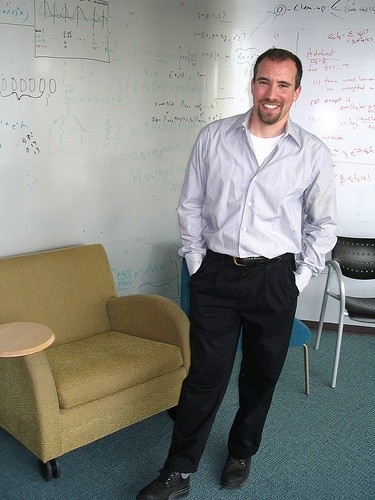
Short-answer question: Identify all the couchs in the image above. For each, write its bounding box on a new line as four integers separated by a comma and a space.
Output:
0, 243, 198, 483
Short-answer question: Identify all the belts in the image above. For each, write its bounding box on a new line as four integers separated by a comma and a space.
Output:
206, 249, 293, 267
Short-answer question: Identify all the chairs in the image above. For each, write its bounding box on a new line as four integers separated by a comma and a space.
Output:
181, 249, 311, 398
315, 236, 375, 390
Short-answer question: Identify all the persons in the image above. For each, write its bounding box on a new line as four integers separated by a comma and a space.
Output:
133, 45, 336, 500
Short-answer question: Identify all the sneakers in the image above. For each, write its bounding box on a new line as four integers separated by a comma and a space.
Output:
135, 472, 191, 500
220, 452, 251, 489
